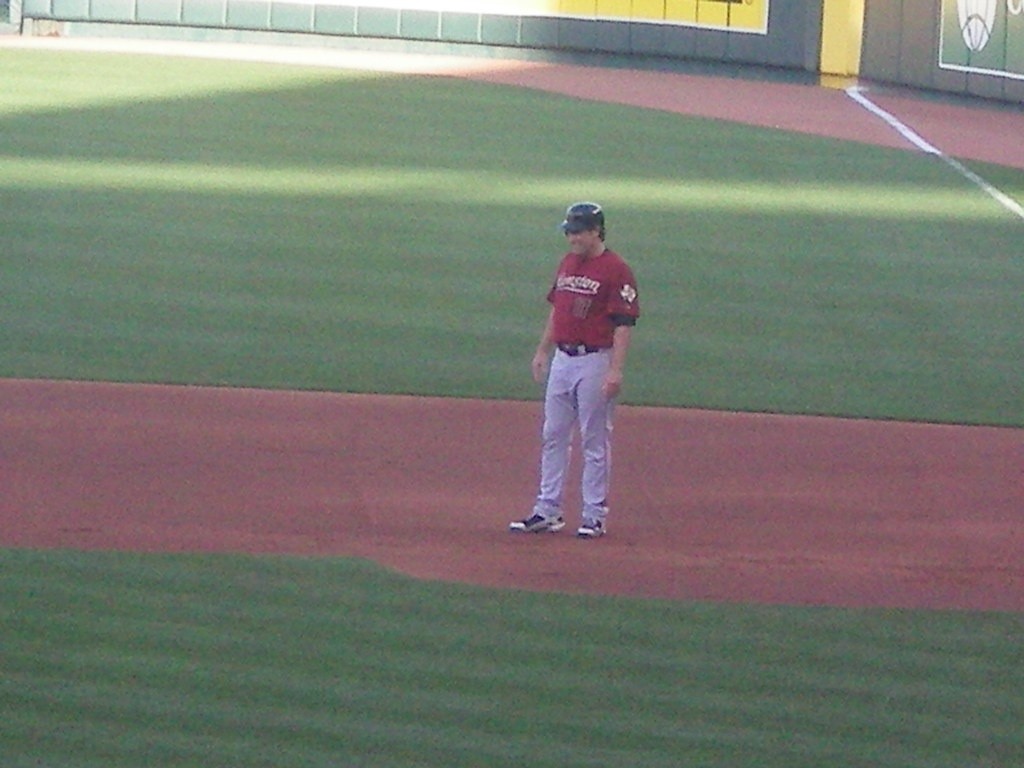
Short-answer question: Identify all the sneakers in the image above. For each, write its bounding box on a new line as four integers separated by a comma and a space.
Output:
509, 511, 566, 534
578, 518, 606, 537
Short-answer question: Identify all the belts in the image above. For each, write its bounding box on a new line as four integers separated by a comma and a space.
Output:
557, 343, 599, 356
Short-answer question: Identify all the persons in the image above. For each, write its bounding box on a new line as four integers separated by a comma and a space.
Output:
509, 203, 640, 539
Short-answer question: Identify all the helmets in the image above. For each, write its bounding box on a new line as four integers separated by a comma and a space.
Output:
561, 202, 604, 232
